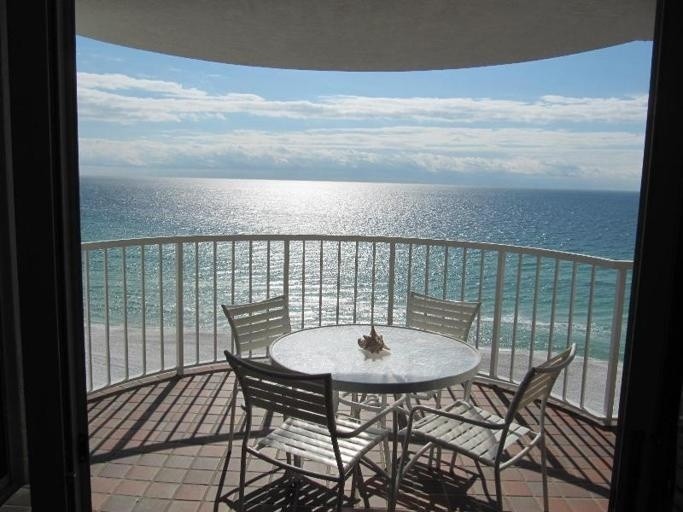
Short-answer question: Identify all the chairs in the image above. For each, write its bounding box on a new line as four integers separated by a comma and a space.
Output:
214, 351, 389, 511
389, 343, 578, 511
389, 291, 483, 425
221, 297, 313, 441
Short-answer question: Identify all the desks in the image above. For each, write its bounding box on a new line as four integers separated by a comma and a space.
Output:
256, 325, 481, 477
